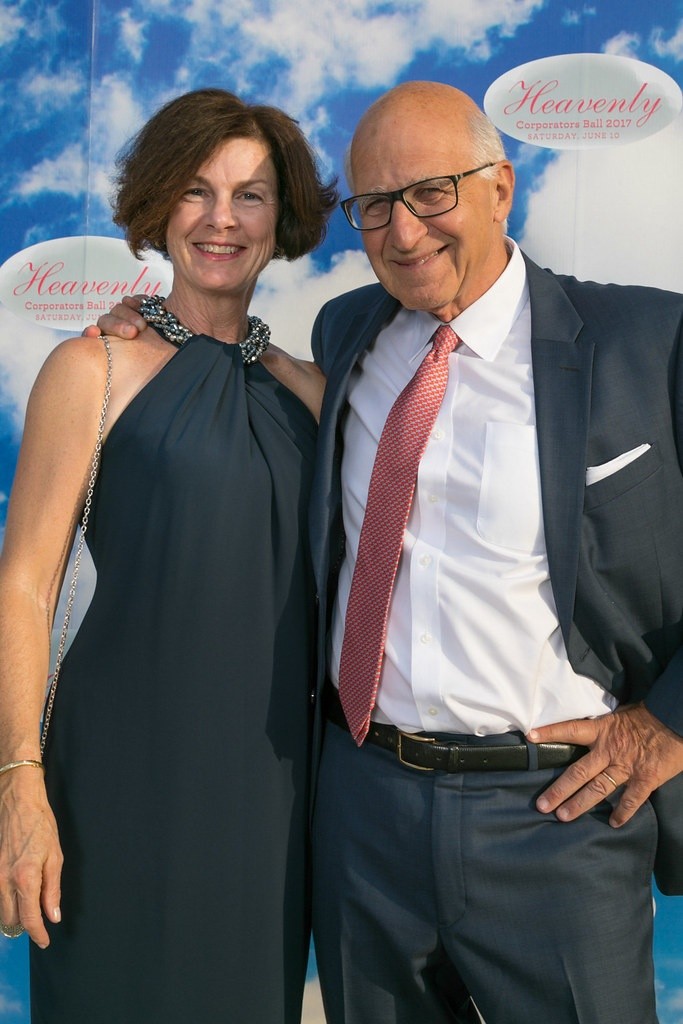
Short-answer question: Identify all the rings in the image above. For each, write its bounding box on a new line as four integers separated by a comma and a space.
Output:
0, 922, 26, 938
603, 770, 617, 789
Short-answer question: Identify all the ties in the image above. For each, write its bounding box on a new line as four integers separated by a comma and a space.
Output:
337, 325, 465, 747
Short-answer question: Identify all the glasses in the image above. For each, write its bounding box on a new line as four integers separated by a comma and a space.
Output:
339, 161, 498, 231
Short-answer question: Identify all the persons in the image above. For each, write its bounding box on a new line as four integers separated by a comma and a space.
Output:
0, 88, 326, 1024
78, 80, 683, 1024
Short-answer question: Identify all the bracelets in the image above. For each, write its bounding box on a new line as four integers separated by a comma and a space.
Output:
0, 760, 46, 777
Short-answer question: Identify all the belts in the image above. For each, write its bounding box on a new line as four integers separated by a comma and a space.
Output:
328, 695, 590, 773
